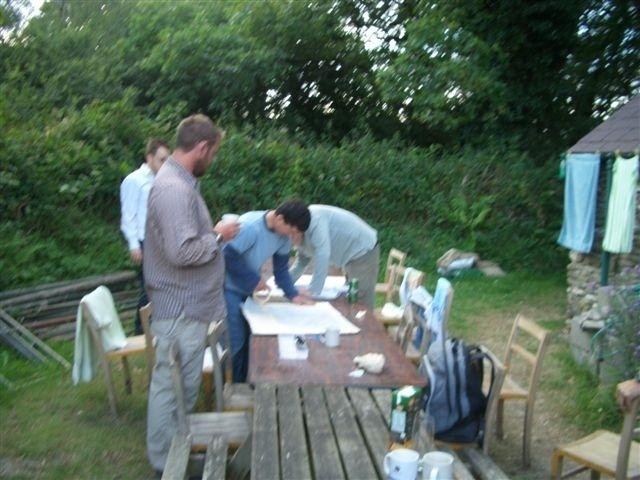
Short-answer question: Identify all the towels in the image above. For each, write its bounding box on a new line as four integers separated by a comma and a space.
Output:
71, 284, 129, 386
556, 150, 602, 254
600, 155, 640, 255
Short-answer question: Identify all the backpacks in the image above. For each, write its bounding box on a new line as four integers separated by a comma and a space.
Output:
412, 337, 495, 445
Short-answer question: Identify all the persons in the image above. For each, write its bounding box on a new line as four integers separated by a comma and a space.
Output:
119, 139, 170, 335
220, 199, 316, 385
144, 114, 242, 480
614, 371, 640, 406
286, 203, 381, 311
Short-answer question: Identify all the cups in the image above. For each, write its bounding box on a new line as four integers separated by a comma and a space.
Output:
421, 451, 455, 480
382, 447, 420, 480
321, 327, 341, 348
222, 213, 239, 227
411, 411, 437, 471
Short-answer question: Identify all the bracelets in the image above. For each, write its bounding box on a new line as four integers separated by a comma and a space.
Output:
211, 232, 224, 245
